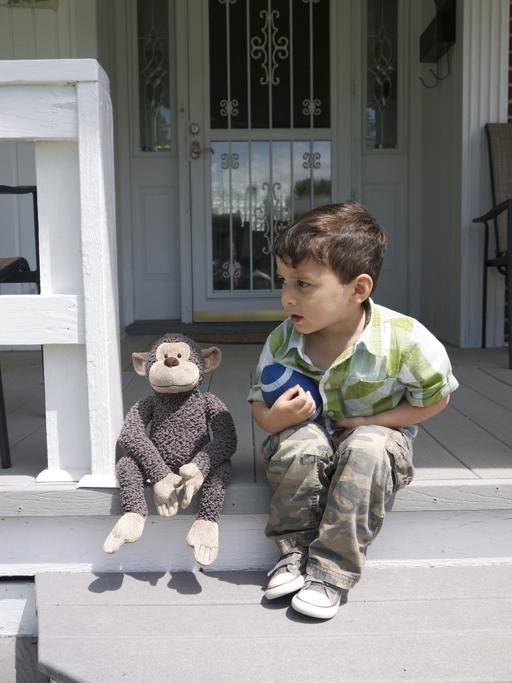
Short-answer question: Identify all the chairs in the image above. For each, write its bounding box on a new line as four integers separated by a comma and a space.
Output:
472, 122, 511, 368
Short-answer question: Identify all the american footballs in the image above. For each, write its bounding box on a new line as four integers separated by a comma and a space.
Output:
261, 364, 322, 421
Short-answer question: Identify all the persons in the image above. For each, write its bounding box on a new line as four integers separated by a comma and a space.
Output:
247, 199, 461, 621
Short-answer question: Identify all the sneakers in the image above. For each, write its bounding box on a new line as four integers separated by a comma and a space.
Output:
264, 552, 307, 600
291, 577, 344, 620
